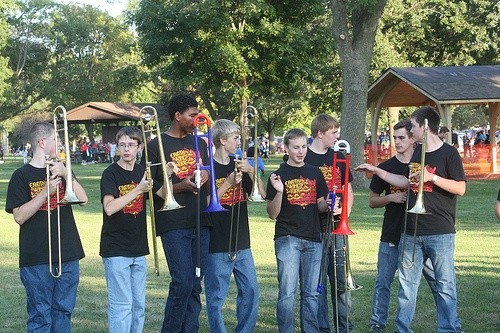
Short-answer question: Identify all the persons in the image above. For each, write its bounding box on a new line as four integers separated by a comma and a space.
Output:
204, 119, 267, 333
99, 126, 178, 333
5, 122, 89, 333
0, 137, 153, 165
246, 134, 339, 159
367, 120, 437, 333
140, 95, 213, 333
353, 106, 467, 333
364, 126, 500, 163
303, 113, 354, 333
266, 129, 341, 333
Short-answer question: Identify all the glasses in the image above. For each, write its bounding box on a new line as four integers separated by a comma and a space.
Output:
117, 142, 139, 149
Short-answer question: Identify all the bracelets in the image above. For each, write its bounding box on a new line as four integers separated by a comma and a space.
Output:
430, 174, 438, 183
168, 174, 172, 180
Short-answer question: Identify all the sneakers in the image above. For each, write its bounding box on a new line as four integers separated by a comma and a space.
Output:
369, 325, 384, 333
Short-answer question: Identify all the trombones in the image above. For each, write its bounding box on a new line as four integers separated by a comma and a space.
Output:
44, 105, 86, 279
401, 118, 432, 269
317, 140, 357, 294
140, 106, 186, 277
193, 114, 229, 279
332, 201, 363, 333
228, 106, 267, 261
